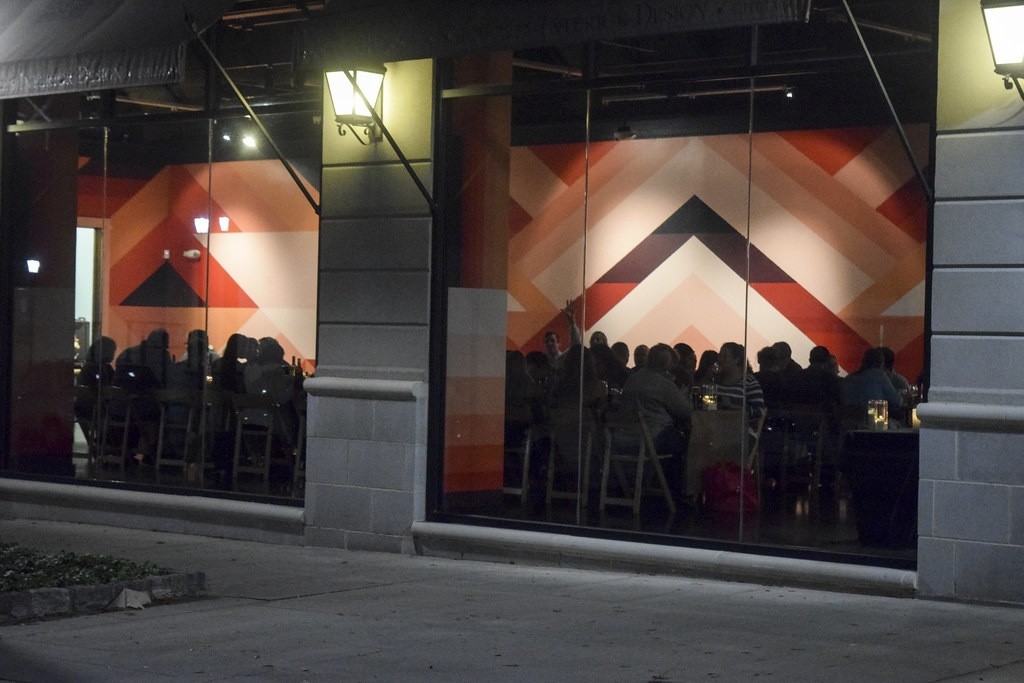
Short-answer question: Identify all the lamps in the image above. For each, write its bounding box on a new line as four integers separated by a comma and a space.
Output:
979, 0, 1024, 100
324, 31, 387, 145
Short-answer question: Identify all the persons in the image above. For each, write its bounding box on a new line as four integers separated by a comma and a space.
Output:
78, 328, 296, 468
505, 299, 913, 490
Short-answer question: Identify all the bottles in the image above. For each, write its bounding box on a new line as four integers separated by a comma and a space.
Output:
289, 355, 315, 391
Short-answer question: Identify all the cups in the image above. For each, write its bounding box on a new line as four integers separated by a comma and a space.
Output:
866, 399, 889, 433
691, 382, 719, 412
912, 409, 921, 432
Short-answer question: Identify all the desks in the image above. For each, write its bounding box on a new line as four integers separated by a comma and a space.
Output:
685, 402, 745, 510
846, 427, 918, 549
768, 407, 837, 487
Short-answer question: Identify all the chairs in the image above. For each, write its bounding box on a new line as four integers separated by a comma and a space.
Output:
71, 374, 306, 495
836, 401, 874, 483
503, 394, 685, 518
747, 406, 767, 505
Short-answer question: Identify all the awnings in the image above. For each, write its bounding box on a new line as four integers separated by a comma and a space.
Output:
0, 0, 319, 214
299, 0, 934, 203
